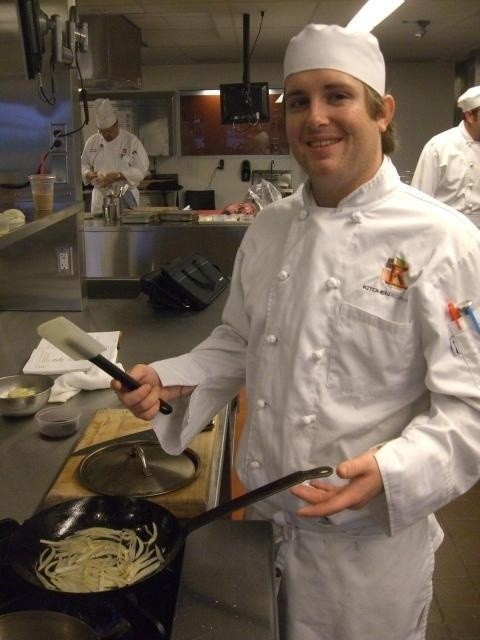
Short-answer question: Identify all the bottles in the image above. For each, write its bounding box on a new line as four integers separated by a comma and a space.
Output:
101, 195, 122, 225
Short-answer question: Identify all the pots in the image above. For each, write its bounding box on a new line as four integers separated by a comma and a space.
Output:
5, 458, 335, 600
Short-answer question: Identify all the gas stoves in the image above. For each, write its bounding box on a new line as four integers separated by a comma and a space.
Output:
1, 518, 281, 640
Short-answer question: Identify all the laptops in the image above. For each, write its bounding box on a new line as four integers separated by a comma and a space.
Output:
186, 190, 215, 210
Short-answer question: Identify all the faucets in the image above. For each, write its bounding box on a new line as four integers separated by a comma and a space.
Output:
269, 159, 277, 178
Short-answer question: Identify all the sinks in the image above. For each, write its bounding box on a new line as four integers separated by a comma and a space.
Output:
275, 188, 294, 198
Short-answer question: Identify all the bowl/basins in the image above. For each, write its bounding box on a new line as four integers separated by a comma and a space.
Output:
1, 371, 83, 440
1, 610, 102, 640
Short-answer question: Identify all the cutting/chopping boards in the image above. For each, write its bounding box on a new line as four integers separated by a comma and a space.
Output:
44, 398, 225, 510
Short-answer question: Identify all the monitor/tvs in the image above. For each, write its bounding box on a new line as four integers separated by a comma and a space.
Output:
16, 0, 48, 79
220, 82, 269, 125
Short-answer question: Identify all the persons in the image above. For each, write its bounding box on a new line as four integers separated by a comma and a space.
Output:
111, 24, 480, 640
409, 86, 480, 231
80, 119, 149, 214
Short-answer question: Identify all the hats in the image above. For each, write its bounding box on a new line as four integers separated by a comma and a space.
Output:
456, 84, 480, 113
281, 22, 388, 99
91, 97, 117, 130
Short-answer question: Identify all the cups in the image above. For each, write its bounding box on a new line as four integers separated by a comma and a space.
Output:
27, 173, 58, 211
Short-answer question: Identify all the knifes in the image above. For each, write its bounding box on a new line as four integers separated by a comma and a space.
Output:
66, 418, 216, 458
